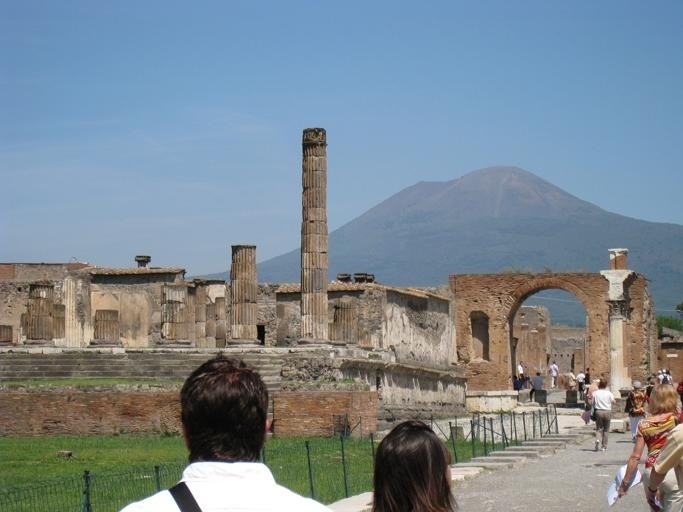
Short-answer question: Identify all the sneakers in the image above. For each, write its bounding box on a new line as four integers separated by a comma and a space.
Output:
595, 440, 608, 450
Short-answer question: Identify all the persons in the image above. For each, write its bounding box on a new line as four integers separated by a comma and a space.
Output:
121, 355, 330, 512
362, 420, 459, 512
513, 359, 683, 512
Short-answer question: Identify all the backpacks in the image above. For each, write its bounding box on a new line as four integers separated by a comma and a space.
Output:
662, 375, 668, 383
